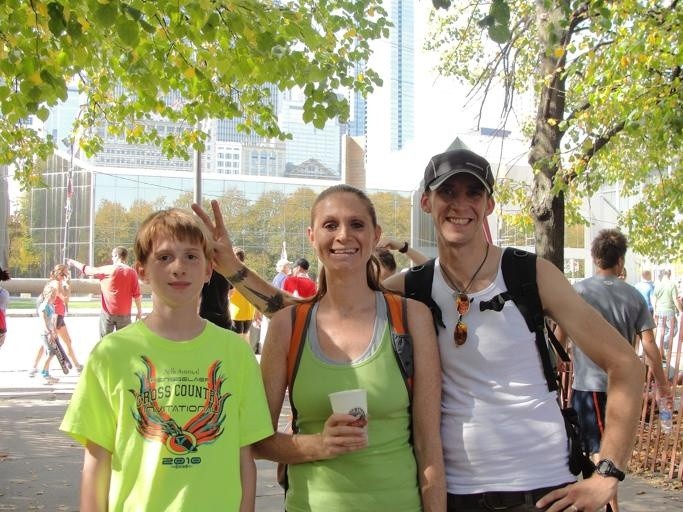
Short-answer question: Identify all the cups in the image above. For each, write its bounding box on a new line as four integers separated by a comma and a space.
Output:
328, 387, 369, 451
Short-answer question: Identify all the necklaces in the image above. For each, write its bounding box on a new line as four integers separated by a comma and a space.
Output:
436, 241, 490, 351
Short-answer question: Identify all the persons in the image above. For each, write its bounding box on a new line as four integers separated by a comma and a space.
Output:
200, 270, 233, 330
188, 150, 649, 511
619, 268, 681, 365
59, 204, 276, 512
34, 284, 70, 380
272, 259, 293, 290
282, 258, 317, 298
550, 229, 674, 512
251, 186, 446, 512
26, 261, 85, 380
227, 248, 255, 338
369, 241, 430, 278
0, 267, 10, 351
64, 246, 144, 338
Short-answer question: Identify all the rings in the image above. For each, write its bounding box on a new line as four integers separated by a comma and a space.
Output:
569, 504, 580, 512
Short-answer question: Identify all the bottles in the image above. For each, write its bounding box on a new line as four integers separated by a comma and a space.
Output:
658, 397, 672, 434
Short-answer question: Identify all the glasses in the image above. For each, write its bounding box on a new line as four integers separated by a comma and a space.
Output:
454, 291, 474, 347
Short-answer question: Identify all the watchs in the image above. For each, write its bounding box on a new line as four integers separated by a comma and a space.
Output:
594, 459, 622, 479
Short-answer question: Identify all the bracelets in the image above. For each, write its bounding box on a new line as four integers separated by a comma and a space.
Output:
79, 265, 86, 274
399, 242, 408, 253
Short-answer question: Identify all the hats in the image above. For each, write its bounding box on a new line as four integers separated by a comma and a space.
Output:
424, 149, 494, 198
